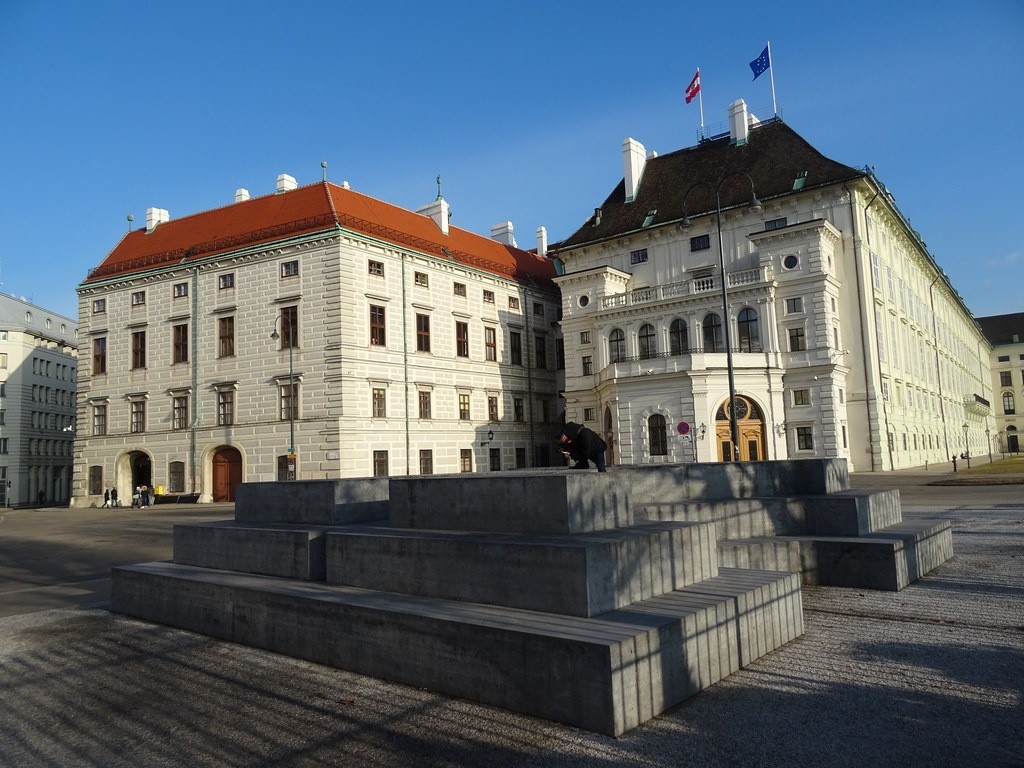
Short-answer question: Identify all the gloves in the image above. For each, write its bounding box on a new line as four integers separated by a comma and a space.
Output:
561, 450, 570, 458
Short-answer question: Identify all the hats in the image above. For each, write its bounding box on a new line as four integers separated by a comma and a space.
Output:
560, 434, 567, 443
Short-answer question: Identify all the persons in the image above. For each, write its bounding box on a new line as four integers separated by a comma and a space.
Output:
102, 485, 154, 508
557, 421, 607, 473
39, 490, 44, 505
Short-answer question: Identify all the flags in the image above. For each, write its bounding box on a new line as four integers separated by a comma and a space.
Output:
749, 46, 770, 81
685, 71, 700, 103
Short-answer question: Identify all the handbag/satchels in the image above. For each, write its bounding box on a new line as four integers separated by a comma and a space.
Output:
112, 500, 116, 506
107, 499, 111, 505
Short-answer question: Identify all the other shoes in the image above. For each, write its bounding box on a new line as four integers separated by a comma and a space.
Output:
569, 462, 589, 469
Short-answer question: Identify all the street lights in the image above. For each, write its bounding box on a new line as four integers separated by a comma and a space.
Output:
270, 309, 296, 481
678, 171, 763, 462
